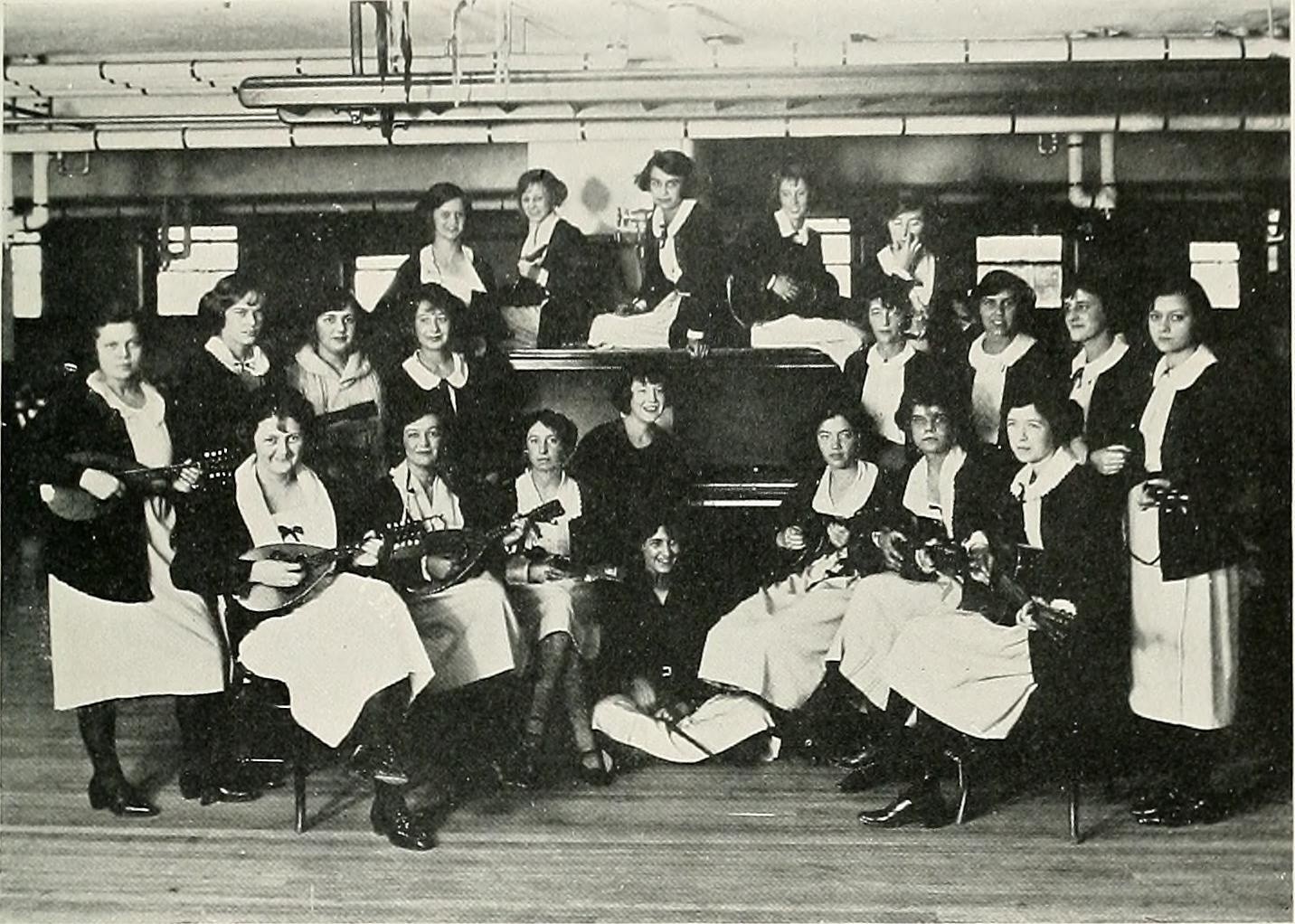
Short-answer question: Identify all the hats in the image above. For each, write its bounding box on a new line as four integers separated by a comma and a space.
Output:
893, 385, 962, 430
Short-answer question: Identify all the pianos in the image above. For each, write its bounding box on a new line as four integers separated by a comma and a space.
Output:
499, 343, 840, 524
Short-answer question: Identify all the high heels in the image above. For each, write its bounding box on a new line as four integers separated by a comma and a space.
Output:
88, 775, 158, 818
178, 769, 260, 806
578, 748, 611, 787
370, 796, 434, 851
519, 715, 548, 749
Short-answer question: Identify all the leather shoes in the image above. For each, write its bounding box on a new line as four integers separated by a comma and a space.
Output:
1129, 791, 1185, 819
856, 791, 946, 828
1137, 797, 1223, 828
827, 744, 888, 795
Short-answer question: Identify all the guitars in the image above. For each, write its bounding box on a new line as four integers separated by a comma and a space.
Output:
880, 506, 965, 581
1095, 424, 1200, 520
960, 536, 1077, 657
38, 445, 250, 524
228, 515, 426, 613
725, 266, 861, 328
313, 399, 382, 447
397, 499, 562, 600
763, 511, 877, 575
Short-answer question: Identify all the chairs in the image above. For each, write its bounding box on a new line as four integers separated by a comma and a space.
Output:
942, 573, 1110, 845
199, 594, 372, 834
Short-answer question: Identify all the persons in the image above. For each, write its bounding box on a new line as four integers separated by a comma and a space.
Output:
177, 269, 1148, 852
587, 151, 723, 358
719, 163, 868, 373
851, 189, 973, 320
11, 308, 258, 819
498, 168, 591, 349
1090, 277, 1250, 828
370, 182, 513, 343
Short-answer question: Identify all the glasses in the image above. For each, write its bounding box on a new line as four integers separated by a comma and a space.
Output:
1062, 303, 1091, 313
909, 413, 955, 426
